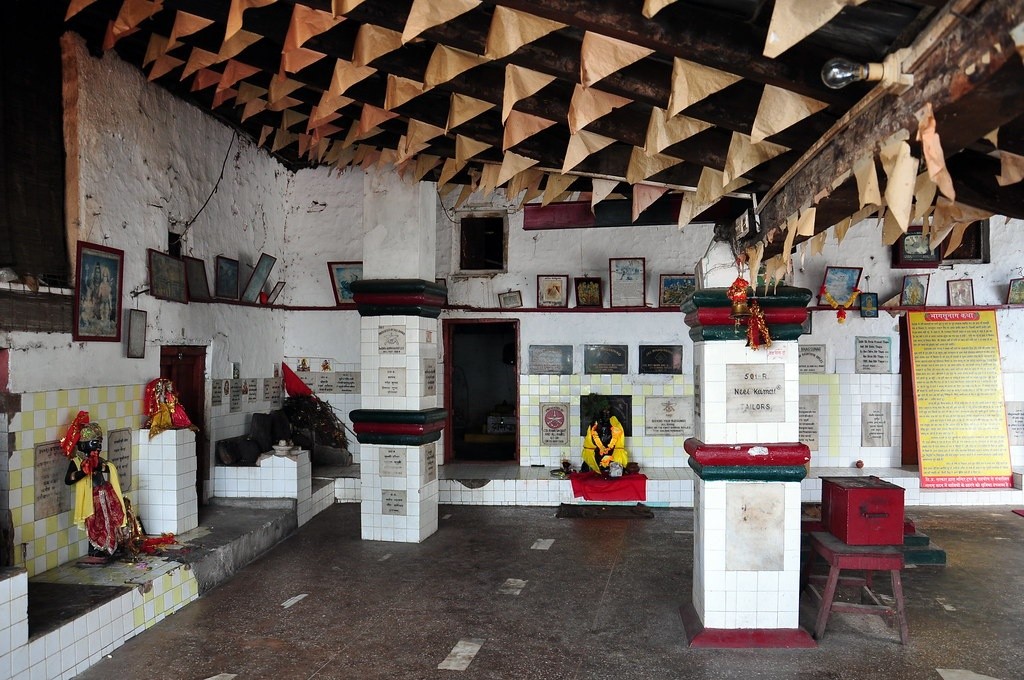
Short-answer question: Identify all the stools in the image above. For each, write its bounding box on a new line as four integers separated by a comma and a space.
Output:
807, 532, 908, 646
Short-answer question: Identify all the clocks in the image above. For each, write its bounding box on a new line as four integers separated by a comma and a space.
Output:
891, 225, 943, 268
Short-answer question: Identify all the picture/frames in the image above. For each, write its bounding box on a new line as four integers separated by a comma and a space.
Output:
72, 240, 1024, 359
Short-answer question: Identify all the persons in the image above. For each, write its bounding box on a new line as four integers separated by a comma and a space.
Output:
80, 269, 112, 328
579, 408, 629, 475
149, 378, 191, 429
64, 423, 125, 556
123, 496, 139, 539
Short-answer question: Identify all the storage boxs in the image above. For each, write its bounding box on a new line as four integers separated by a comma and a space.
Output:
819, 476, 905, 544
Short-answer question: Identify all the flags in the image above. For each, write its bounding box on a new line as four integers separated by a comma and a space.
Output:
282, 363, 314, 398
